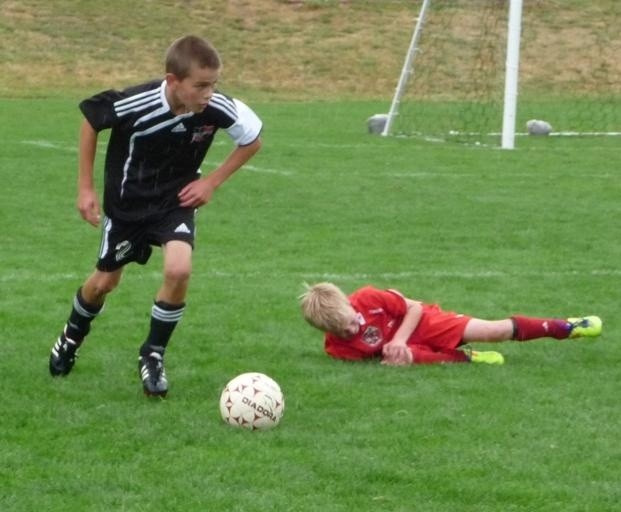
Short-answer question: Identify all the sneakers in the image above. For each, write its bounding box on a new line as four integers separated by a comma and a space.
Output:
48, 322, 83, 378
137, 343, 170, 397
566, 315, 604, 338
463, 348, 506, 365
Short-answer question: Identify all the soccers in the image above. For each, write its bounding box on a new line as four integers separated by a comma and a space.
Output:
220, 371, 286, 430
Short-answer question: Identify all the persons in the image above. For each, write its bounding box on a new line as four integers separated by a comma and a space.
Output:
297, 281, 603, 364
46, 33, 264, 398
